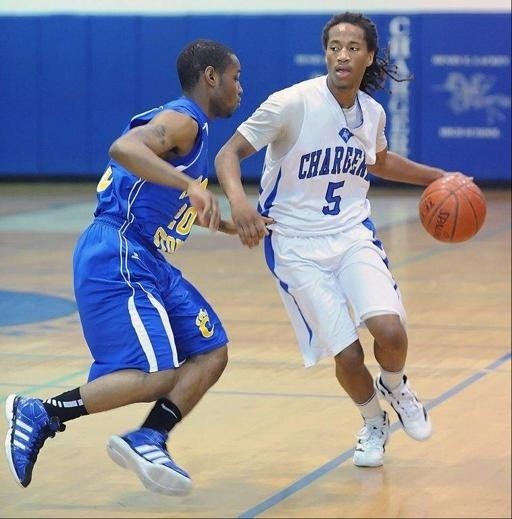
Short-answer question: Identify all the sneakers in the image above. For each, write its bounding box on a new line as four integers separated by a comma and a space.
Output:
106, 429, 194, 497
374, 372, 432, 443
353, 409, 392, 468
4, 391, 66, 489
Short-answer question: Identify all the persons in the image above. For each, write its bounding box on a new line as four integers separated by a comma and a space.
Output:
211, 12, 479, 469
3, 38, 276, 496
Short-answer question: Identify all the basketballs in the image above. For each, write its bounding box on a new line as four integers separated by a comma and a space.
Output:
418, 174, 486, 243
